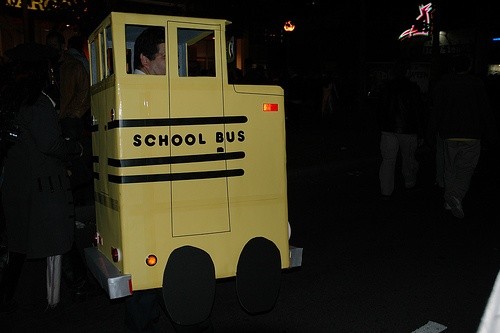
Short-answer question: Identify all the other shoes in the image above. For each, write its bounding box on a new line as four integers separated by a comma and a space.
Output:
444, 191, 465, 220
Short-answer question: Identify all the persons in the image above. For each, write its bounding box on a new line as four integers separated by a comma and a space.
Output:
1, 31, 105, 312
127, 27, 165, 77
244, 44, 374, 238
433, 56, 496, 219
375, 63, 425, 202
